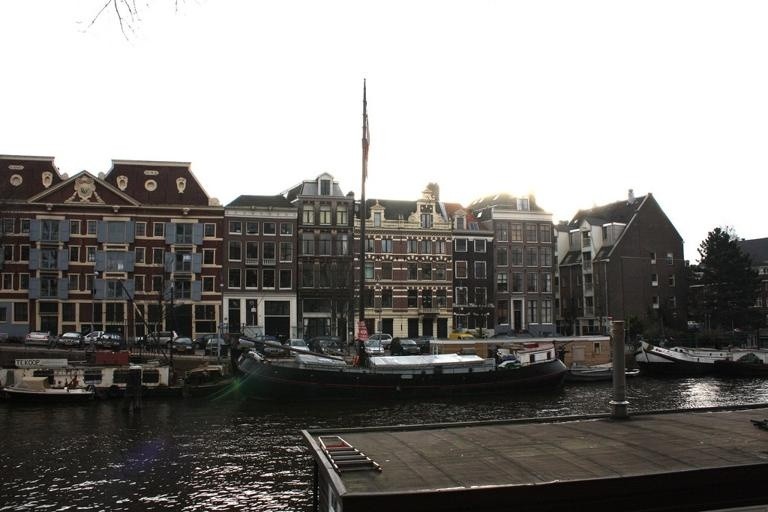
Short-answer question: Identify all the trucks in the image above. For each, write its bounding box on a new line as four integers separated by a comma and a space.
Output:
449, 333, 476, 340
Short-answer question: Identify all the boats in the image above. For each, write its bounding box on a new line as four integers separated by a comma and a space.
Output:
1, 344, 235, 400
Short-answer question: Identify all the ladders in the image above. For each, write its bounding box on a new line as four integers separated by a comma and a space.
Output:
318, 436, 382, 476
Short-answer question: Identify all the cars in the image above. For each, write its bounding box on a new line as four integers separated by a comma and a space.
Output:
23, 329, 234, 358
